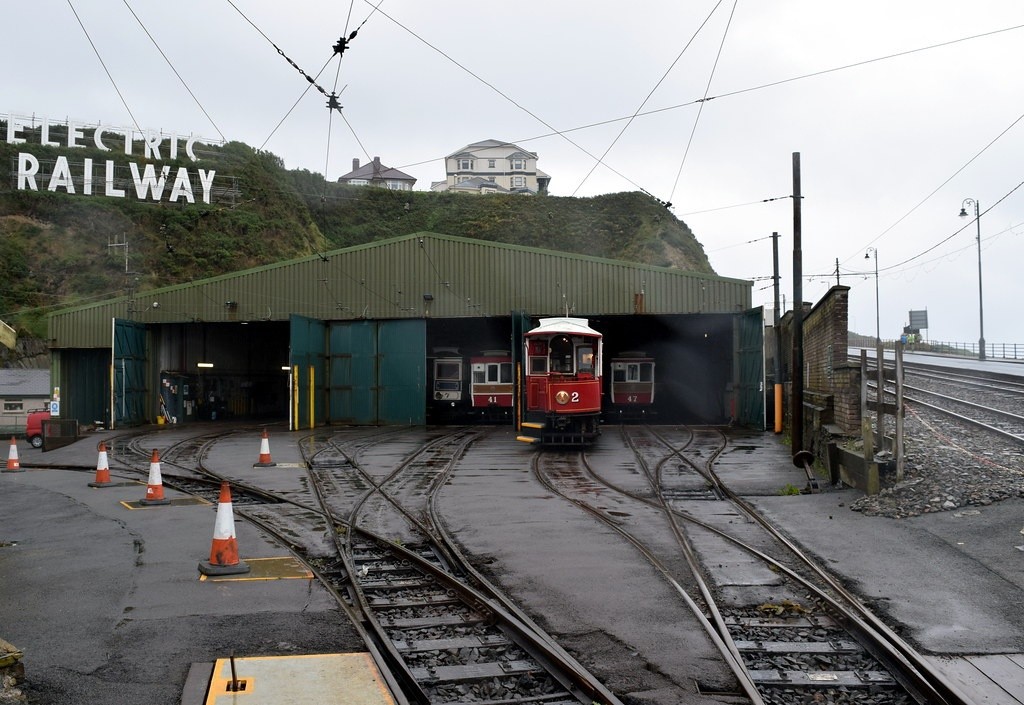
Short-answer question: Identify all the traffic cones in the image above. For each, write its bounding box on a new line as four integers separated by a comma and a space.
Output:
87, 438, 118, 488
140, 447, 171, 505
1, 436, 26, 473
196, 479, 250, 576
253, 428, 276, 467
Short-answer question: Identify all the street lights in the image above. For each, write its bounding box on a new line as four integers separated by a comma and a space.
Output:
865, 246, 881, 348
959, 196, 986, 360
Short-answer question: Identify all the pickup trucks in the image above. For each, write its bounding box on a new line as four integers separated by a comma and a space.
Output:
27, 407, 51, 449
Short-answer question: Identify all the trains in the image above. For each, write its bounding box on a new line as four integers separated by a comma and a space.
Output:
470, 349, 517, 427
523, 317, 604, 453
610, 350, 659, 425
